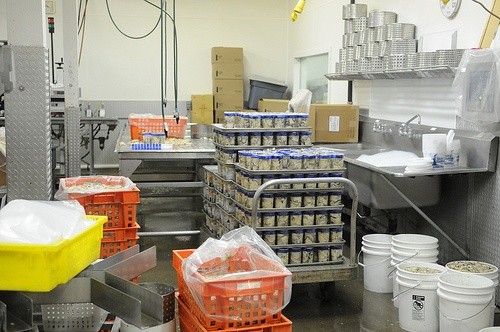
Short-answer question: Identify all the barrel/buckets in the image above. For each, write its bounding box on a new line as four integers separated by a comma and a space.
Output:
445, 260, 498, 290
390, 261, 448, 332
388, 234, 440, 309
434, 272, 500, 332
357, 234, 393, 294
360, 289, 394, 332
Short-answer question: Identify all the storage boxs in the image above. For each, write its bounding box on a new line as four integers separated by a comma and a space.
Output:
257, 96, 291, 113
211, 46, 244, 124
189, 94, 214, 125
0, 212, 107, 293
59, 176, 140, 258
172, 246, 293, 332
129, 113, 188, 142
248, 79, 288, 110
309, 104, 360, 144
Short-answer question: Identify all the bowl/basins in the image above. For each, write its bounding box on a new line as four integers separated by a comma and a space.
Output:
421, 134, 462, 168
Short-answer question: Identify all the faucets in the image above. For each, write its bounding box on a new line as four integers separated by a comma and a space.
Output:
398, 114, 422, 136
370, 119, 393, 136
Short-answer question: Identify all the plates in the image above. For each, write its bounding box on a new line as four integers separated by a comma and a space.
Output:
404, 158, 433, 174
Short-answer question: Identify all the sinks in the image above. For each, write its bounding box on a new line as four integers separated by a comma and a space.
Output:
321, 142, 384, 152
345, 149, 441, 209
